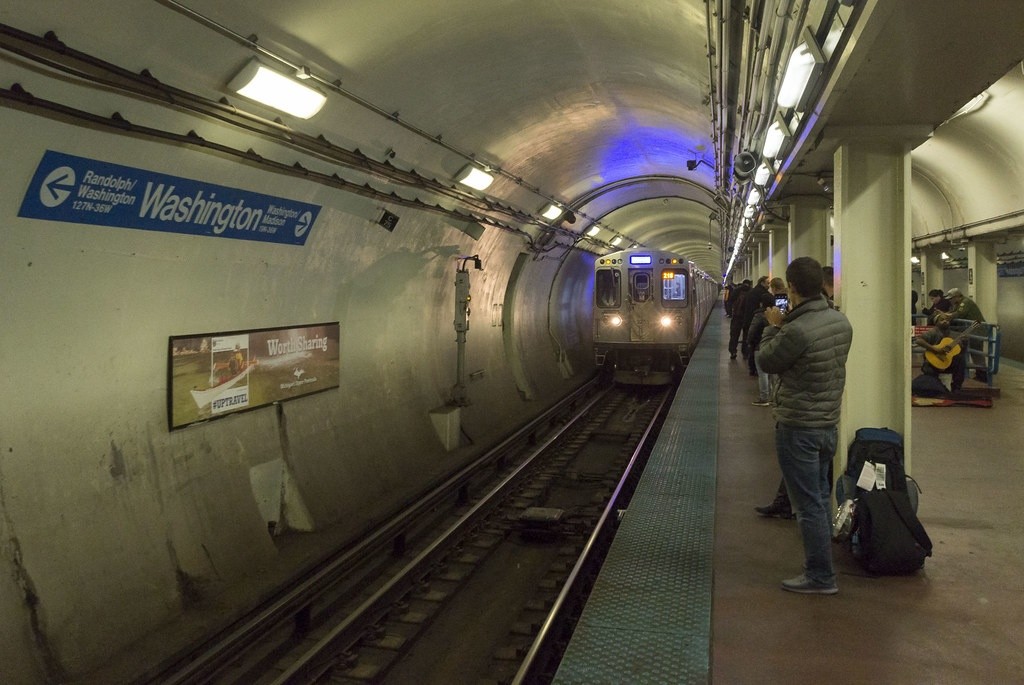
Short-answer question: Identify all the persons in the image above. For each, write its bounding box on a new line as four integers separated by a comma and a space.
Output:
228, 344, 244, 377
912, 288, 990, 396
725, 256, 854, 593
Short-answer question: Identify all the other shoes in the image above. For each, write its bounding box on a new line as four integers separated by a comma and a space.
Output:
752, 400, 769, 406
731, 352, 737, 359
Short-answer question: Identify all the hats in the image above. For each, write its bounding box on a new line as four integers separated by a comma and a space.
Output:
945, 288, 961, 300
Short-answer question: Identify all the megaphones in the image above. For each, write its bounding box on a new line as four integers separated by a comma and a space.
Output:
735, 150, 759, 174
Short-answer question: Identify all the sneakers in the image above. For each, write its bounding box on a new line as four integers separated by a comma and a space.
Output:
781, 573, 838, 594
753, 499, 793, 519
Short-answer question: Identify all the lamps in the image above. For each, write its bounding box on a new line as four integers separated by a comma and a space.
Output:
609, 236, 622, 246
452, 162, 495, 191
776, 26, 827, 112
763, 111, 792, 161
222, 55, 327, 120
723, 156, 775, 284
584, 225, 600, 237
538, 203, 562, 220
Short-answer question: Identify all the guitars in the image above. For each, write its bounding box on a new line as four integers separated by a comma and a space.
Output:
922, 319, 982, 373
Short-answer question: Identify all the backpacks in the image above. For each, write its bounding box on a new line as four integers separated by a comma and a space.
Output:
834, 427, 933, 578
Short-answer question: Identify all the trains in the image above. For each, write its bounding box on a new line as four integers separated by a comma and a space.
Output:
592, 244, 719, 378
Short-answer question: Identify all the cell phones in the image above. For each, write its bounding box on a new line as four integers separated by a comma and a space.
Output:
774, 293, 788, 317
